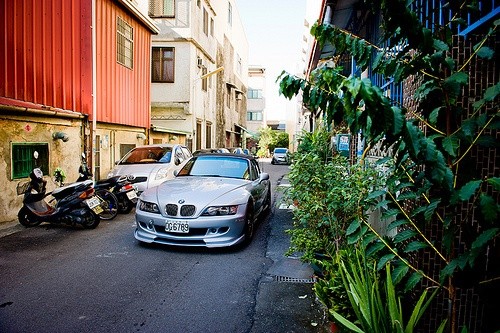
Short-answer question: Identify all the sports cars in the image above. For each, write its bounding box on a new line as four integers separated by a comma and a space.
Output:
134, 154, 271, 251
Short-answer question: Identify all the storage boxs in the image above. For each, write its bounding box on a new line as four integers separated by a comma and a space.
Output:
34, 193, 56, 213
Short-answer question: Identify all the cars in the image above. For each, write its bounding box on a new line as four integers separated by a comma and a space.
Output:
272, 148, 288, 165
193, 147, 253, 158
108, 145, 188, 193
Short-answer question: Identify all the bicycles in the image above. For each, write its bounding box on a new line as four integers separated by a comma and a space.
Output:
53, 168, 119, 220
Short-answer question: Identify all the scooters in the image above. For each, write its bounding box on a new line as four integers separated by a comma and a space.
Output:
17, 151, 104, 229
76, 152, 136, 214
